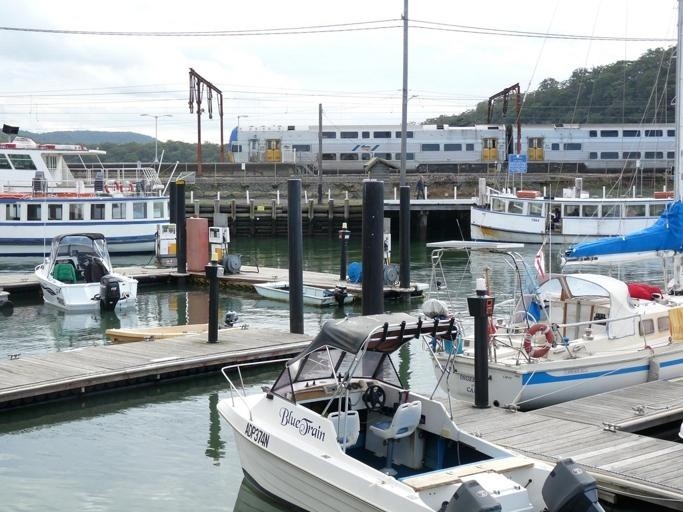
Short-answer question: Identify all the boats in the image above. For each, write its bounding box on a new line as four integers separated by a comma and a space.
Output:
0, 123, 178, 257
215, 312, 604, 512
0, 289, 13, 310
41, 300, 138, 352
103, 311, 236, 344
252, 279, 354, 307
29, 231, 137, 306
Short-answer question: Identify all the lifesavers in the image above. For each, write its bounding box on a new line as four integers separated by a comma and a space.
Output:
445, 330, 464, 354
105, 180, 119, 196
523, 323, 554, 358
120, 181, 134, 195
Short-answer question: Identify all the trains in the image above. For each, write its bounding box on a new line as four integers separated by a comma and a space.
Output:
225, 124, 676, 172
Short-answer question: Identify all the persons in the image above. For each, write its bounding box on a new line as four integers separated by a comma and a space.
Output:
415, 176, 426, 200
551, 208, 560, 229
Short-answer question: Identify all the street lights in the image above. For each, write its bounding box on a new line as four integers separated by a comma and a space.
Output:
235, 114, 250, 163
405, 91, 420, 107
141, 114, 173, 161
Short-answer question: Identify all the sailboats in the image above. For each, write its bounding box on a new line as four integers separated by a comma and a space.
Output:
468, 0, 683, 245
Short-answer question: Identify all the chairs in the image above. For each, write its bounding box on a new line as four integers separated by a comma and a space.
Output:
370, 401, 421, 478
498, 292, 535, 330
52, 263, 76, 283
330, 408, 360, 453
